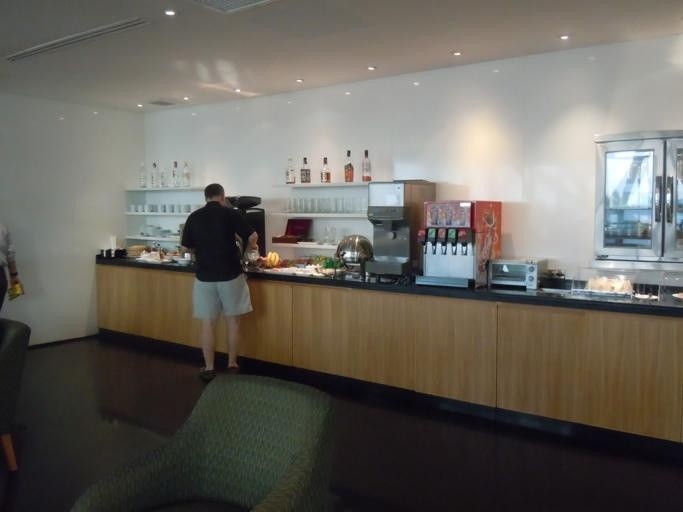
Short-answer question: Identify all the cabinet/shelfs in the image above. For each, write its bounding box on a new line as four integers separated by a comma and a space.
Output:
269, 181, 372, 249
124, 186, 210, 244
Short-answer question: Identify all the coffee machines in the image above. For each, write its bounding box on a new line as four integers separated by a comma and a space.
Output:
362, 178, 439, 286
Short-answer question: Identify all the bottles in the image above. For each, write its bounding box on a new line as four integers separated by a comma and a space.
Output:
321, 157, 332, 183
478, 226, 495, 271
362, 149, 372, 182
344, 150, 354, 182
300, 156, 310, 183
139, 162, 191, 189
284, 158, 296, 184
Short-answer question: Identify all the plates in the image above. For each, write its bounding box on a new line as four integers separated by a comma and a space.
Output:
297, 240, 321, 245
130, 245, 193, 267
138, 223, 180, 239
262, 263, 345, 280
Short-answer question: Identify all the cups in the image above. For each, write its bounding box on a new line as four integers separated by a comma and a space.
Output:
129, 202, 202, 214
283, 197, 367, 214
605, 218, 651, 240
325, 225, 338, 244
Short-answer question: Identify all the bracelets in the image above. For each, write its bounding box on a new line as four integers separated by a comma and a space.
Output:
9, 272, 17, 277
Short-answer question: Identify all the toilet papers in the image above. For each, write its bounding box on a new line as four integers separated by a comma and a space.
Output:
110, 234, 117, 258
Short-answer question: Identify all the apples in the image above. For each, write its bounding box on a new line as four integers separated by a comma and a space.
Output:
256, 255, 266, 266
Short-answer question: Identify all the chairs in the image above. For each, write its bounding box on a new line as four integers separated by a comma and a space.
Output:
0, 317, 31, 472
52, 372, 335, 512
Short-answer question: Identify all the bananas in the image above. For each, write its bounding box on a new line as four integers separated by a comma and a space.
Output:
267, 251, 279, 267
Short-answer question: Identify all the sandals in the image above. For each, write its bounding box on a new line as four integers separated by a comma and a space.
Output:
198, 364, 217, 382
225, 365, 241, 375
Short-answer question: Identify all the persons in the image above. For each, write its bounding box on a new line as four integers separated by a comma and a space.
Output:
0, 224, 21, 309
182, 184, 259, 383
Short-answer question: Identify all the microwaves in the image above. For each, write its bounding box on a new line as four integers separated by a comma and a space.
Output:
487, 256, 550, 293
